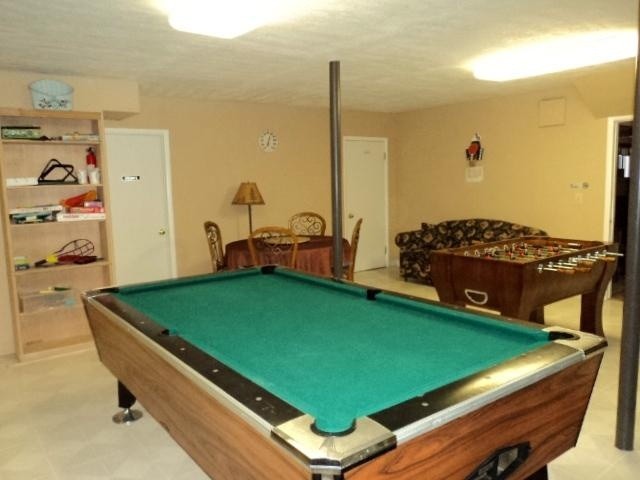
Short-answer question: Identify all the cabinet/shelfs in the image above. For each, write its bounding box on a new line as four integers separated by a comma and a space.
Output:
0, 109, 114, 360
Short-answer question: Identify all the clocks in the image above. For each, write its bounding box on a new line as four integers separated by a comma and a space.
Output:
258, 129, 280, 152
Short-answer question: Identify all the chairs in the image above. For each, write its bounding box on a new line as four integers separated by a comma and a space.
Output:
203, 212, 364, 283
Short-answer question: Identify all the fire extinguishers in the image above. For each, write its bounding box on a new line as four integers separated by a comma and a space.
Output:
87, 146, 96, 184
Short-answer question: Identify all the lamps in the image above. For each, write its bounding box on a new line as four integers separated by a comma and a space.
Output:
231, 180, 267, 238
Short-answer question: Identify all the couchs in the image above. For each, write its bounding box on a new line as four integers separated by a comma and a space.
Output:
396, 219, 551, 290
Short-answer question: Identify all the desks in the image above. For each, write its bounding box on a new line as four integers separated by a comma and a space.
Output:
78, 265, 607, 480
